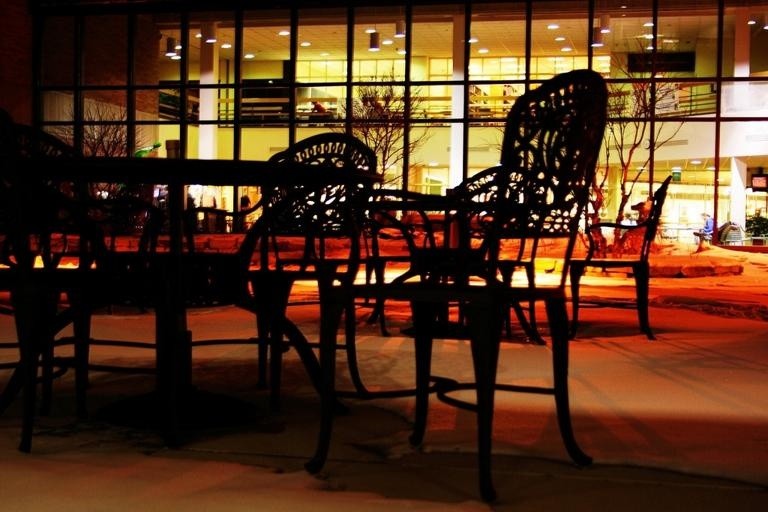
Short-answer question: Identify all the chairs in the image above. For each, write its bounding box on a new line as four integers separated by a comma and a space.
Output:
0, 69, 672, 503
693, 222, 738, 244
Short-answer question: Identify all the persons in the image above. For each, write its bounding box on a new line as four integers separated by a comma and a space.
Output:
238, 194, 260, 224
631, 197, 654, 225
699, 213, 713, 234
311, 101, 327, 113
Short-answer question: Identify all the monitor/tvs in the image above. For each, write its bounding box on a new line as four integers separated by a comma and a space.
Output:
752, 174, 768, 193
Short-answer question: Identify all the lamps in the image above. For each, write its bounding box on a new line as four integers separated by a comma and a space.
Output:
370, 8, 379, 51
600, 15, 612, 33
164, 30, 177, 57
590, 17, 604, 47
395, 7, 406, 38
